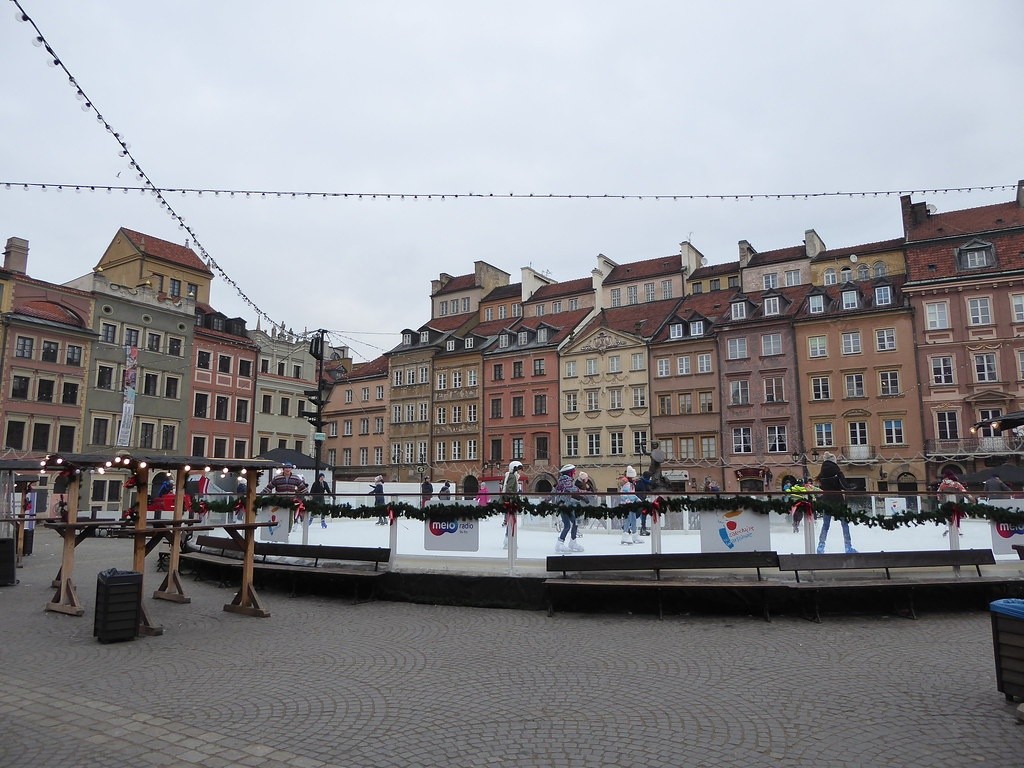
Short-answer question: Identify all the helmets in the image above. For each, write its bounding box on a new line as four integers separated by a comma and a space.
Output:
509, 461, 523, 474
559, 464, 575, 474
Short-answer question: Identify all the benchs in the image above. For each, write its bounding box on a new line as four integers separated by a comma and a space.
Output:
543, 548, 1024, 622
183, 533, 390, 600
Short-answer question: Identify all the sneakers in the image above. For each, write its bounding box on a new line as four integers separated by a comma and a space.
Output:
621, 532, 634, 545
554, 537, 572, 553
308, 517, 313, 526
568, 538, 584, 552
632, 532, 645, 543
321, 519, 327, 528
503, 537, 518, 549
381, 518, 388, 526
375, 518, 384, 525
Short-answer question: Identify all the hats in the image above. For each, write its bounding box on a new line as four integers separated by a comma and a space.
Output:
626, 466, 637, 478
643, 471, 652, 480
318, 473, 325, 478
481, 482, 486, 487
445, 482, 450, 487
823, 451, 837, 463
283, 462, 294, 468
579, 471, 589, 480
375, 474, 383, 482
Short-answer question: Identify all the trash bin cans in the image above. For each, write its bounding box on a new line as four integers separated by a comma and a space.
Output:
93, 572, 143, 643
990, 598, 1024, 702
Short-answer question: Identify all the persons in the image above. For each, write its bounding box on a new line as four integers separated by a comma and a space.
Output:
984, 472, 1013, 499
158, 480, 175, 498
260, 461, 312, 534
369, 475, 388, 526
236, 478, 247, 520
422, 476, 433, 508
635, 471, 653, 536
620, 465, 645, 545
554, 464, 588, 553
501, 465, 521, 549
475, 482, 489, 506
816, 451, 857, 553
937, 469, 976, 537
574, 472, 589, 502
438, 482, 450, 500
308, 473, 336, 529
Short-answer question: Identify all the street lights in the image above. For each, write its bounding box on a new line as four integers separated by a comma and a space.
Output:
308, 328, 348, 483
791, 448, 819, 484
483, 458, 501, 477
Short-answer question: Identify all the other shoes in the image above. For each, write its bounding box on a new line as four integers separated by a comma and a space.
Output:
817, 546, 824, 554
943, 526, 949, 537
846, 547, 858, 553
958, 527, 963, 537
